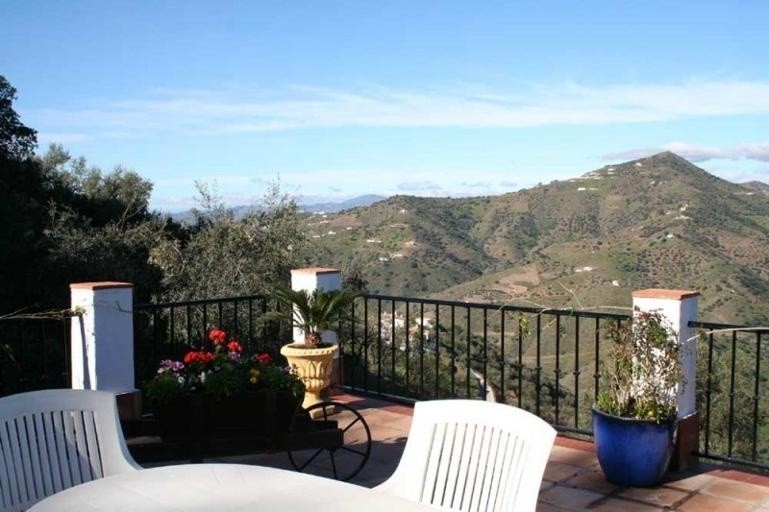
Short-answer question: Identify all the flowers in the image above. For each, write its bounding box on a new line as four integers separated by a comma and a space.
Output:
138, 326, 311, 403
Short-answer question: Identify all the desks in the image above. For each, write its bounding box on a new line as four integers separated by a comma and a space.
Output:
17, 458, 467, 512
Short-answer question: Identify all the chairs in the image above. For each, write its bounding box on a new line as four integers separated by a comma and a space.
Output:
367, 395, 557, 512
0, 387, 156, 512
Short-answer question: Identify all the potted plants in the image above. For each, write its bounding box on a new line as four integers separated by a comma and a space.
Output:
262, 279, 371, 419
590, 300, 691, 489
